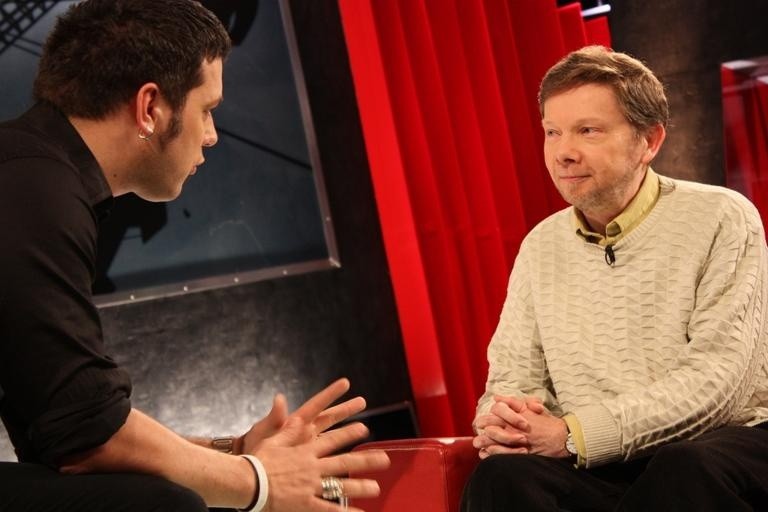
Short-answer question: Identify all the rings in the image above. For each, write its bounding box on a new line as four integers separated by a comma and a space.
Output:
320, 476, 345, 501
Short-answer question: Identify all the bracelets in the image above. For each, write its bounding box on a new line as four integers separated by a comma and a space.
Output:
237, 451, 270, 511
212, 434, 236, 454
561, 429, 578, 462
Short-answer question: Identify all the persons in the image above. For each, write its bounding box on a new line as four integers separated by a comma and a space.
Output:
456, 43, 768, 511
0, 0, 395, 510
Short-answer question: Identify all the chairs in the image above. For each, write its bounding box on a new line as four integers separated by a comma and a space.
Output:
349, 437, 479, 512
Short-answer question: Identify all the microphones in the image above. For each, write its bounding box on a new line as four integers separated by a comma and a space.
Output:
604, 244, 617, 266
